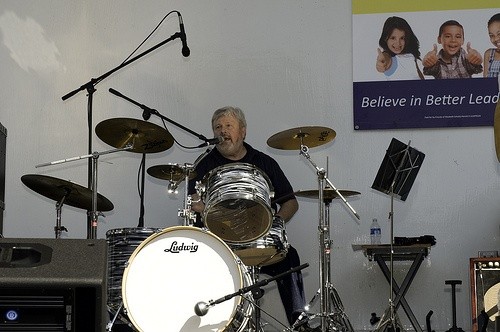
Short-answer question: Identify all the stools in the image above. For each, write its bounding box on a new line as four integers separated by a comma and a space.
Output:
365, 244, 429, 332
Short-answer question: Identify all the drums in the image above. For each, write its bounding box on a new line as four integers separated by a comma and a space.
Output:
229, 213, 290, 268
204, 163, 277, 244
120, 225, 254, 332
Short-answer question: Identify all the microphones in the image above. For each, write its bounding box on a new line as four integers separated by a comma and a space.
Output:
177, 12, 190, 58
197, 135, 225, 148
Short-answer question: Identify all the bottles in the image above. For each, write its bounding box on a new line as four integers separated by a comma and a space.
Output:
370, 218, 381, 244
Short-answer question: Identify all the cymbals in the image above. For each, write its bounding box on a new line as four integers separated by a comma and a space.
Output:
95, 116, 175, 155
146, 162, 186, 182
20, 172, 115, 214
266, 125, 337, 152
293, 188, 362, 202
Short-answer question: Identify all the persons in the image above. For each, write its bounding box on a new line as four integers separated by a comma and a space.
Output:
188, 106, 311, 331
422, 19, 484, 79
376, 16, 426, 81
484, 13, 500, 78
